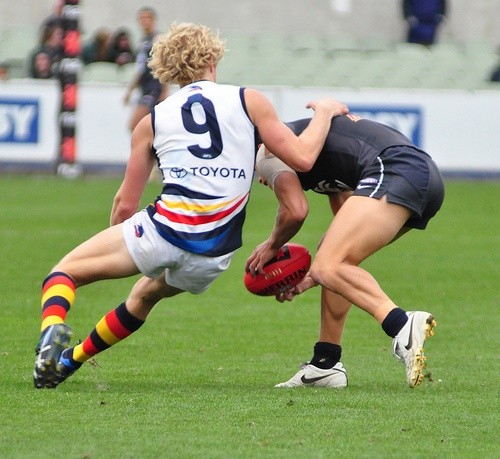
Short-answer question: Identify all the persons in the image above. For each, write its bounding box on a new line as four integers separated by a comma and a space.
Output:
33, 21, 349, 390
399, 0, 448, 48
244, 115, 444, 389
23, 0, 168, 181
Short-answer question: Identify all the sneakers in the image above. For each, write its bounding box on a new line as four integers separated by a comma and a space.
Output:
273, 360, 348, 389
391, 311, 437, 388
33, 325, 73, 388
45, 344, 77, 389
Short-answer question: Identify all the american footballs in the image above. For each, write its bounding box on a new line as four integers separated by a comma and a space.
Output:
245, 243, 312, 296
